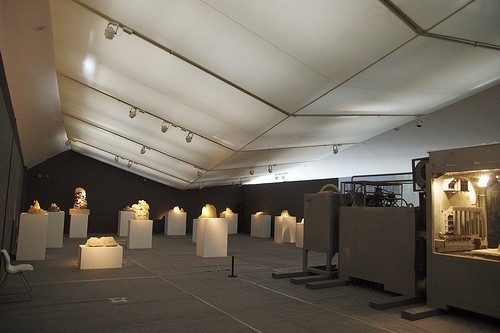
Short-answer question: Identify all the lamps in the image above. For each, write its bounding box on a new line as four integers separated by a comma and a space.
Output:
104, 21, 119, 39
268, 166, 272, 174
186, 132, 193, 142
197, 168, 203, 177
129, 106, 137, 118
140, 146, 146, 155
250, 167, 255, 176
161, 121, 170, 133
333, 144, 338, 154
417, 117, 423, 127
127, 161, 133, 167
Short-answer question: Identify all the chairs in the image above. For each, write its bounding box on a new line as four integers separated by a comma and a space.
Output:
0, 248, 33, 299
